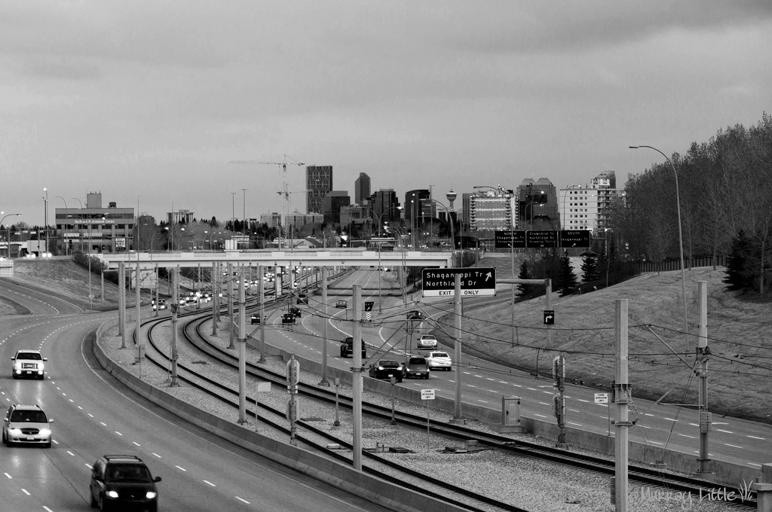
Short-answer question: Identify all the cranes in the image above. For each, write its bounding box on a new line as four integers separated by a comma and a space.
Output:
276, 183, 313, 249
227, 152, 306, 239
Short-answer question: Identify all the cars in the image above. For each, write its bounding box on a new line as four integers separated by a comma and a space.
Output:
289, 307, 302, 318
401, 355, 430, 379
406, 311, 426, 319
369, 359, 403, 382
370, 266, 391, 272
281, 313, 296, 323
416, 334, 438, 348
250, 313, 267, 326
423, 350, 452, 371
336, 300, 348, 308
151, 266, 312, 312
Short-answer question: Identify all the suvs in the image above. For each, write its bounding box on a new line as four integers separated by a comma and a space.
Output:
340, 337, 366, 358
2, 402, 55, 448
10, 349, 48, 379
89, 453, 162, 512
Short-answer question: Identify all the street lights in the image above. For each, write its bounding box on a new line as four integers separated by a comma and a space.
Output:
240, 189, 248, 249
628, 145, 690, 353
62, 214, 257, 308
230, 192, 238, 250
349, 203, 404, 315
429, 184, 436, 251
419, 198, 456, 268
471, 182, 610, 231
382, 221, 408, 250
41, 188, 49, 257
0, 213, 22, 228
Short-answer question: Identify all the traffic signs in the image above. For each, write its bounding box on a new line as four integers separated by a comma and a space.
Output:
558, 231, 589, 248
422, 268, 496, 298
526, 230, 558, 248
494, 231, 526, 248
